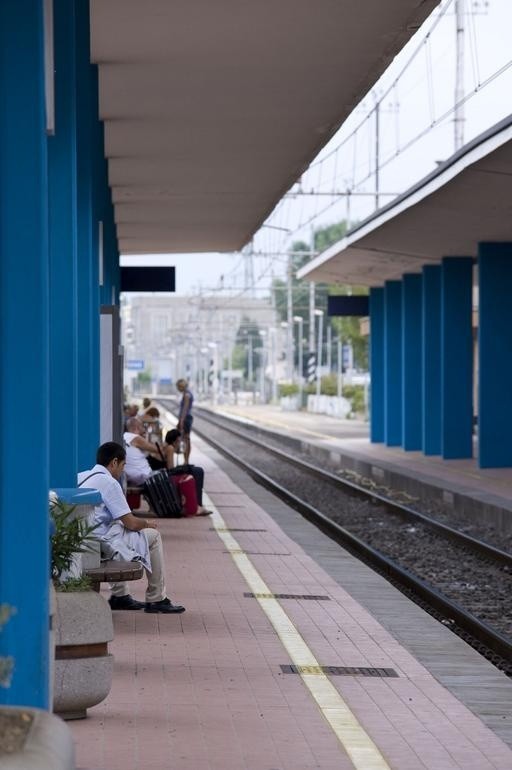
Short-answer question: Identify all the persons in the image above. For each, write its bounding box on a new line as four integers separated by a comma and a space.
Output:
77, 442, 185, 614
122, 379, 214, 517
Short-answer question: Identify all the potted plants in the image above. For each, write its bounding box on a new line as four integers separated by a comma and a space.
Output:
41, 496, 117, 721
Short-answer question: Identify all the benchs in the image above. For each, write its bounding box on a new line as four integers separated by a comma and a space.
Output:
79, 557, 142, 594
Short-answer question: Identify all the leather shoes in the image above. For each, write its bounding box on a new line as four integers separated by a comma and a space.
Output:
109, 594, 146, 610
143, 595, 185, 614
197, 505, 213, 516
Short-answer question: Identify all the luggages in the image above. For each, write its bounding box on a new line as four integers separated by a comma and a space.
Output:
171, 475, 198, 517
145, 471, 183, 520
